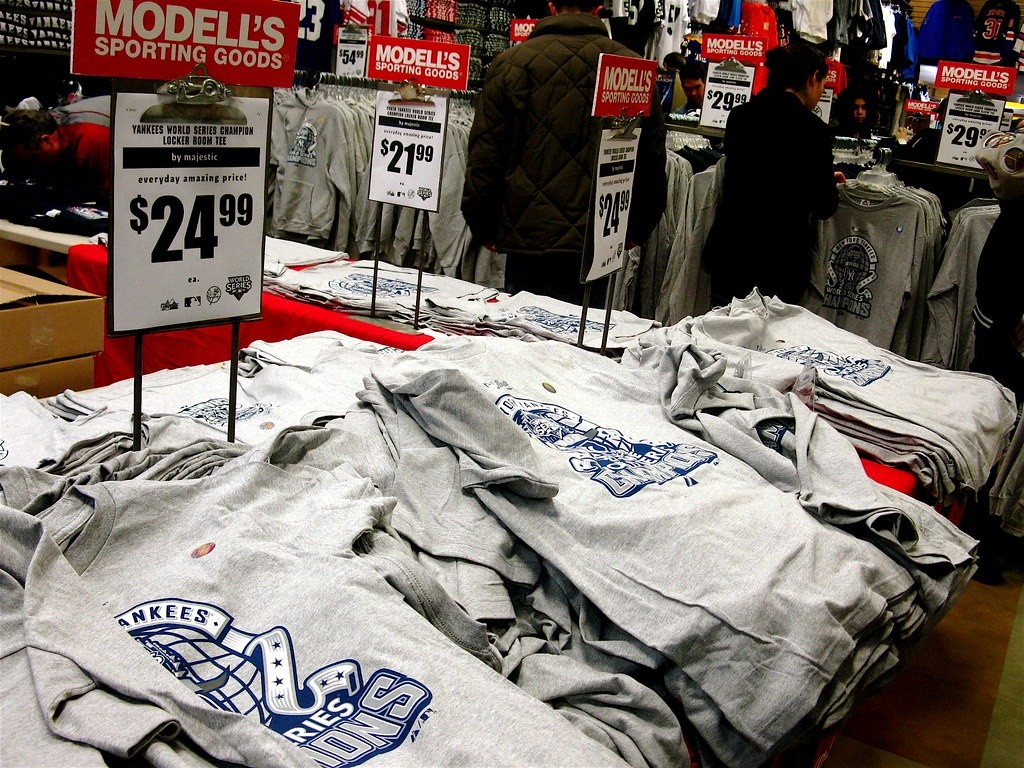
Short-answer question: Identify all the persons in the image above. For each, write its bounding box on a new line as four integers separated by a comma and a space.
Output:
829, 86, 876, 140
459, 1, 669, 309
699, 43, 846, 310
894, 96, 948, 154
970, 130, 1024, 409
673, 59, 707, 114
1, 95, 112, 199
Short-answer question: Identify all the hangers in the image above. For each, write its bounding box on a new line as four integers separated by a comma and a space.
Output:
271, 70, 483, 129
830, 136, 913, 200
666, 113, 711, 154
879, 0, 910, 20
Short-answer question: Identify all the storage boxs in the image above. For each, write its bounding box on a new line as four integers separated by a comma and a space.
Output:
0, 353, 95, 399
0, 266, 108, 371
